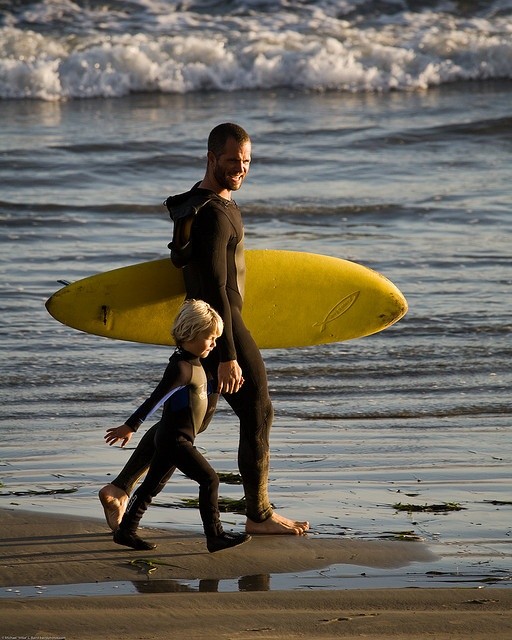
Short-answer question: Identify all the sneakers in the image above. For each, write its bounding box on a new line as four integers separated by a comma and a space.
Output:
113, 526, 157, 551
206, 531, 252, 554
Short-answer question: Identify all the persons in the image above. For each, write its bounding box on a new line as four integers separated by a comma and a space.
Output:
105, 298, 253, 553
97, 121, 310, 536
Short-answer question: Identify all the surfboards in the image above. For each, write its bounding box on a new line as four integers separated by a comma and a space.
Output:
44, 249, 408, 350
